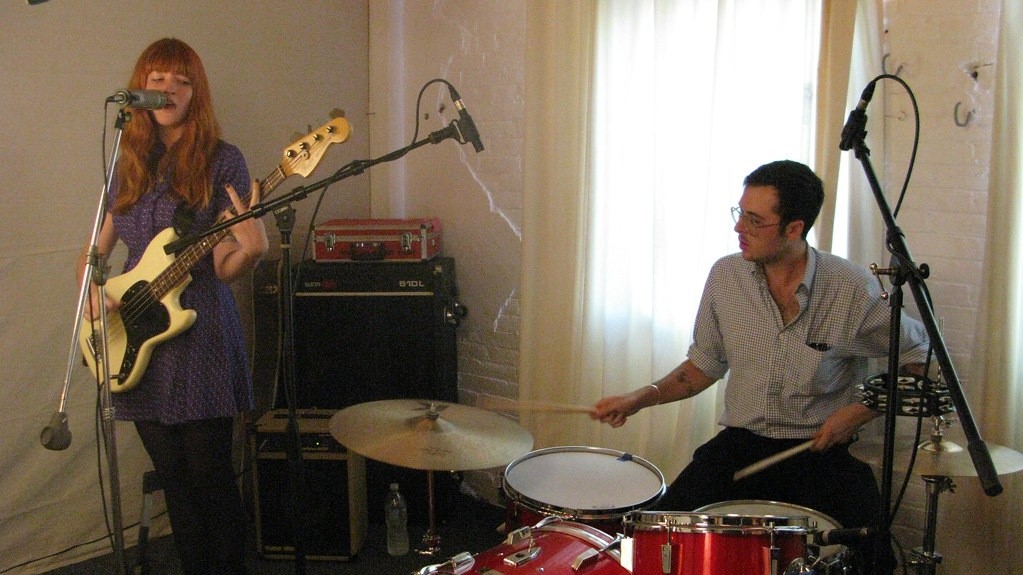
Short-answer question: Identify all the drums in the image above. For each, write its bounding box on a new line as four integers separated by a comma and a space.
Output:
623, 510, 811, 574
501, 446, 667, 536
412, 517, 622, 575
693, 498, 846, 575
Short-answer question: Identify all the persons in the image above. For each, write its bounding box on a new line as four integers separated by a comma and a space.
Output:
76, 37, 269, 575
588, 159, 937, 575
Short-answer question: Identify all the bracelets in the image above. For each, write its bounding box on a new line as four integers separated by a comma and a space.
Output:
649, 384, 662, 405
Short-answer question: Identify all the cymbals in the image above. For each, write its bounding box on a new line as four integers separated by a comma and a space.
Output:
328, 399, 535, 472
848, 434, 1023, 477
855, 373, 957, 417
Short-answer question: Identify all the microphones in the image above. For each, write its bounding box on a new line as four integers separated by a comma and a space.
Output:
448, 84, 484, 153
814, 526, 875, 546
839, 79, 877, 152
108, 89, 166, 110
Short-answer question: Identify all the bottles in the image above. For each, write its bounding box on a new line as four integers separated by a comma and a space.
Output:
385, 484, 410, 556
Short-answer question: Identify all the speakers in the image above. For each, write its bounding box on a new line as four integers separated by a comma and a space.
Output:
286, 257, 463, 522
252, 408, 369, 560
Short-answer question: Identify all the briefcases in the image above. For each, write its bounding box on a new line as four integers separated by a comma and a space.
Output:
310, 216, 442, 263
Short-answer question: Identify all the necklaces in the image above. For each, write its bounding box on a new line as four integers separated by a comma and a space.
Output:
157, 160, 165, 183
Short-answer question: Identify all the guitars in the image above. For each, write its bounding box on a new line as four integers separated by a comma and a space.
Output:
77, 108, 352, 395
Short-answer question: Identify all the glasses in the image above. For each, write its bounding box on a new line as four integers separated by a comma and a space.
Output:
731, 204, 784, 237
